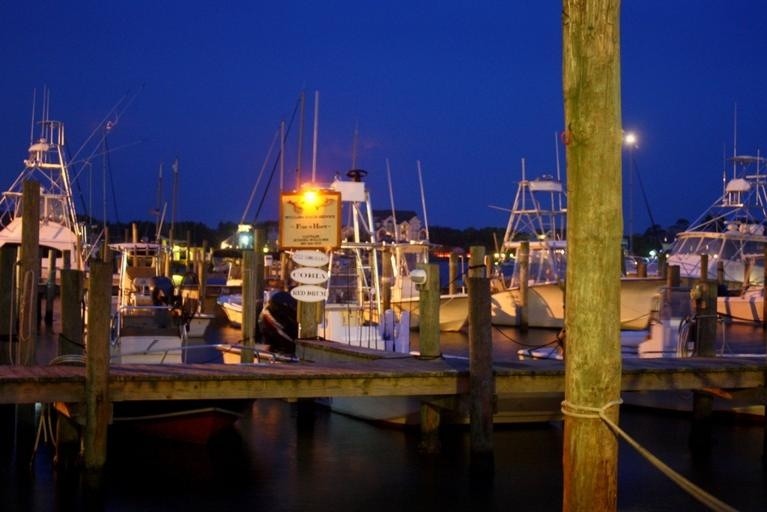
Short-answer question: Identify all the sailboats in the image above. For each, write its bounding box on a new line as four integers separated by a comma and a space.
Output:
457, 124, 663, 333
642, 96, 765, 323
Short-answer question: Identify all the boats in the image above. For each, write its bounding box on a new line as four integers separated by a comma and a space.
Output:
516, 318, 765, 362
108, 306, 301, 365
0, 85, 470, 332
